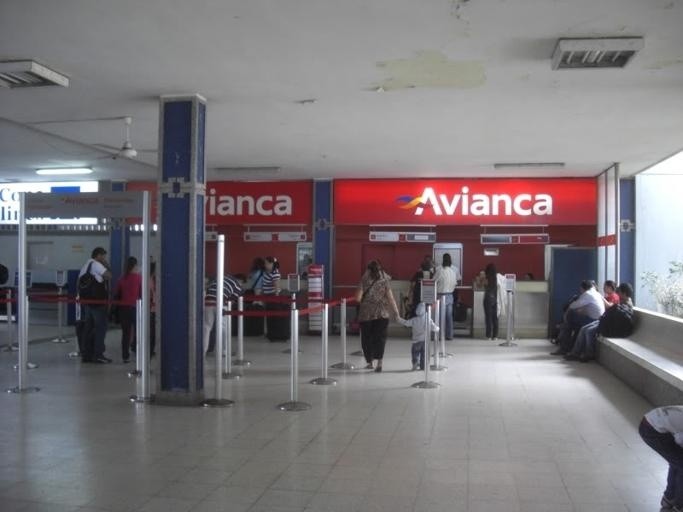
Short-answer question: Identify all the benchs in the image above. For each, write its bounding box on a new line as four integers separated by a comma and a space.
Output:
595, 306, 682, 410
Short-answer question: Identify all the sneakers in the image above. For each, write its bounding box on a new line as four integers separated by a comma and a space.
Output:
660, 495, 682, 512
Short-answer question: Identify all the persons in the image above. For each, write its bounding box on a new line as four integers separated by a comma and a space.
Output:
202, 272, 246, 355
113, 257, 142, 362
638, 404, 682, 511
249, 258, 265, 304
263, 256, 279, 338
395, 302, 439, 370
148, 262, 157, 374
356, 260, 398, 372
76, 247, 112, 364
406, 253, 634, 362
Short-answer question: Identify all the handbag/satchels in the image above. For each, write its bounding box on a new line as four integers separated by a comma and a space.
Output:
78, 272, 107, 309
243, 289, 254, 303
350, 316, 359, 332
454, 301, 466, 322
602, 304, 634, 337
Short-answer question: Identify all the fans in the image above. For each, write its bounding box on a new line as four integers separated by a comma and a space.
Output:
90, 113, 160, 166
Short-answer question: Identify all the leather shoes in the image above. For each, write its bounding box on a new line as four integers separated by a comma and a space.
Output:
84, 355, 112, 363
